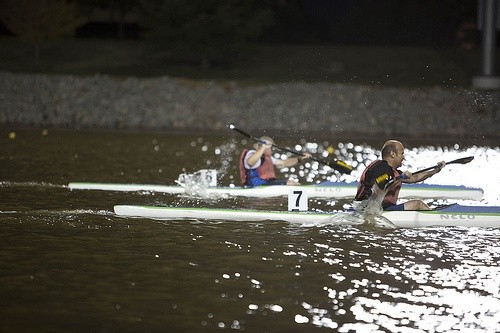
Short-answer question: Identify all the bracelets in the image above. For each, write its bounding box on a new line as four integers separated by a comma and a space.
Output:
298, 157, 302, 163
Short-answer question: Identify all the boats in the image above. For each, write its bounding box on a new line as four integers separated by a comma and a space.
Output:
67, 182, 484, 200
114, 202, 500, 229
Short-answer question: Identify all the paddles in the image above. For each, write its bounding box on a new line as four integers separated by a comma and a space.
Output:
222, 123, 353, 174
374, 155, 476, 190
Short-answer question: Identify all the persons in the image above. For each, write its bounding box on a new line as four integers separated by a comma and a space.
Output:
355, 141, 445, 212
241, 135, 313, 187
